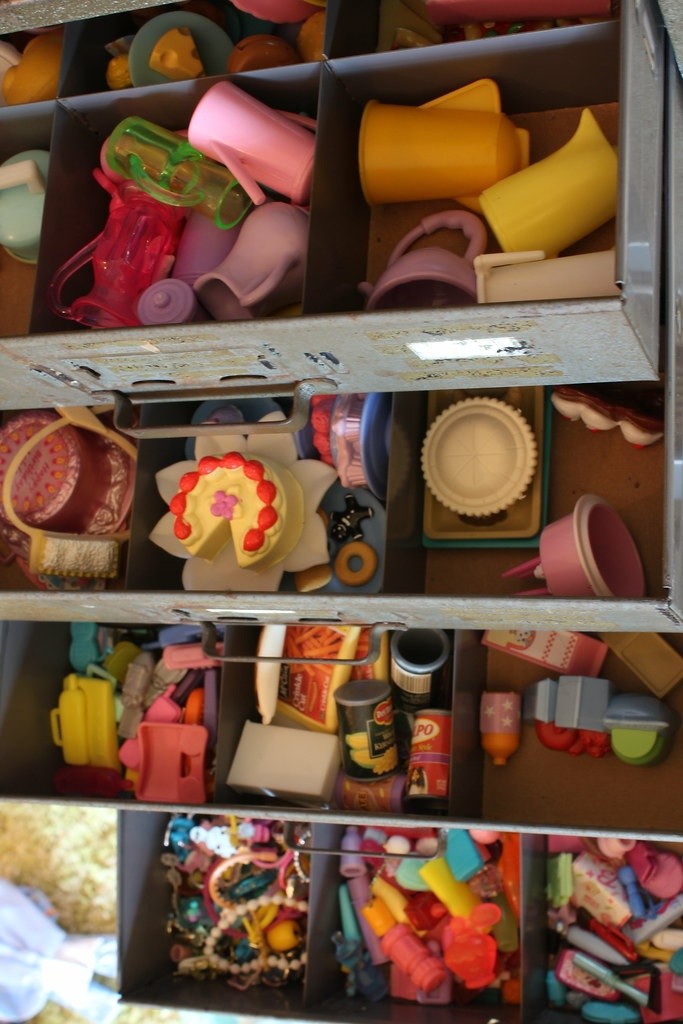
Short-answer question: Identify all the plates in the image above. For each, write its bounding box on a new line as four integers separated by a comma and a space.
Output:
359, 391, 391, 498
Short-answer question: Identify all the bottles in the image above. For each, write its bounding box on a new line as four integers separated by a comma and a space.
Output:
479, 692, 520, 765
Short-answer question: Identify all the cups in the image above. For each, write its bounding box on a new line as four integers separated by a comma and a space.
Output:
49, 79, 316, 325
357, 78, 620, 312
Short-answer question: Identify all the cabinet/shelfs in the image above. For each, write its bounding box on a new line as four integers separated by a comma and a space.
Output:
0, 0, 683, 1024
